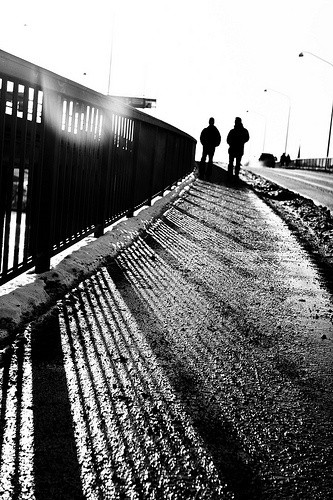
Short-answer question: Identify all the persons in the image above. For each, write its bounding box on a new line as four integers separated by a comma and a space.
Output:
200, 117, 221, 167
279, 153, 286, 168
285, 154, 291, 169
226, 117, 249, 179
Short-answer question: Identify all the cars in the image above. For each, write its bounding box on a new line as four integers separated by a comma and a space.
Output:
258, 153, 275, 168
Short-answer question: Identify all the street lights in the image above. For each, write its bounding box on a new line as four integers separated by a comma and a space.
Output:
246, 110, 267, 153
298, 50, 333, 158
264, 88, 292, 156
